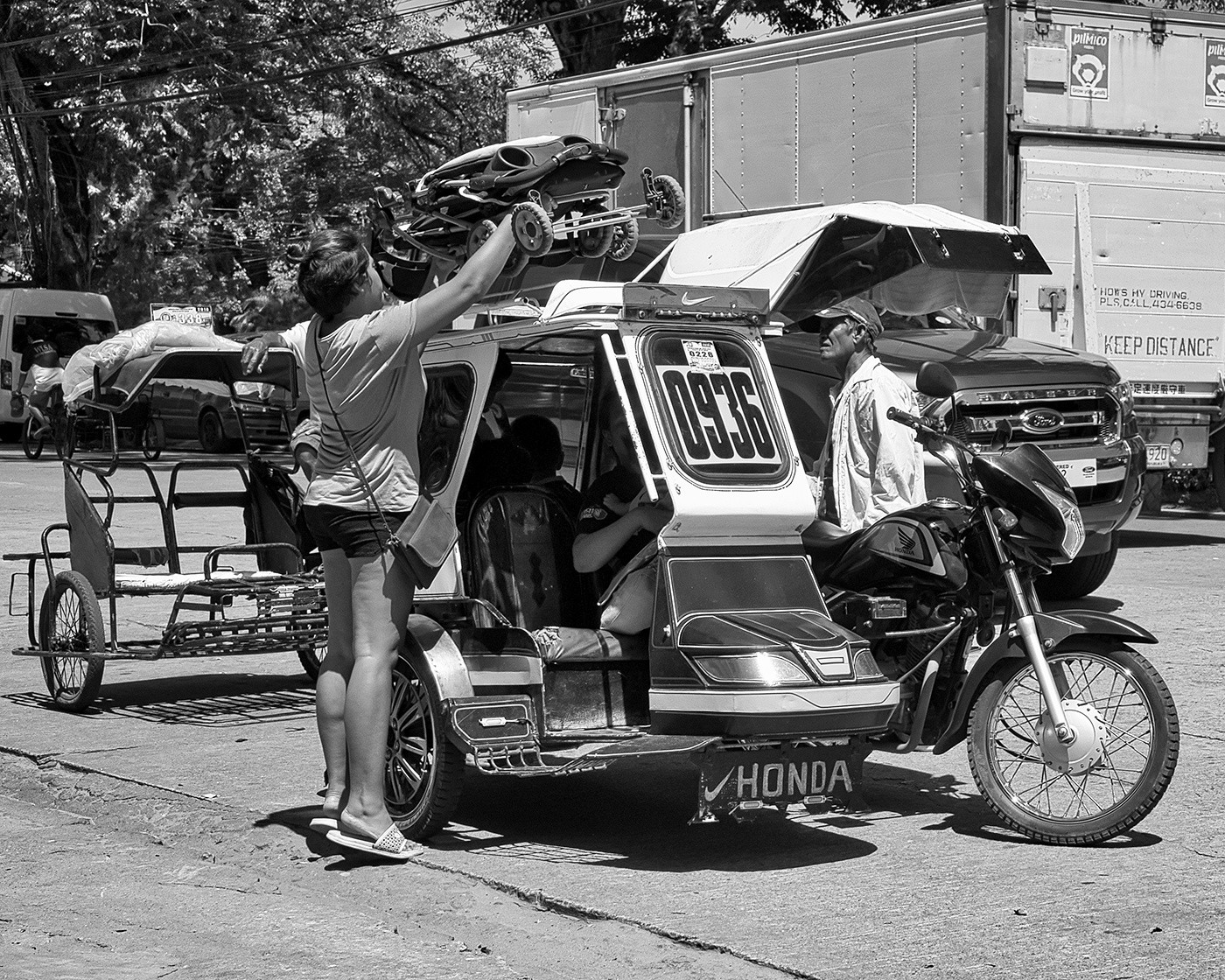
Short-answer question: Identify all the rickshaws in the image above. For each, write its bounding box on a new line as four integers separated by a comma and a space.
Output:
380, 200, 1181, 849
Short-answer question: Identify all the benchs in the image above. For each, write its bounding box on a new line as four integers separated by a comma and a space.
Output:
61, 457, 291, 600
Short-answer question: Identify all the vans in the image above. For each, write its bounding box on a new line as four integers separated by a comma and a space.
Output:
0, 282, 117, 442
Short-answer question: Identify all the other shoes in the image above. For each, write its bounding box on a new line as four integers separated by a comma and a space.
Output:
34, 425, 50, 440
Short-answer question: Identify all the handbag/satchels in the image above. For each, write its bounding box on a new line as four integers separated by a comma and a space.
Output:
597, 540, 659, 635
385, 488, 461, 590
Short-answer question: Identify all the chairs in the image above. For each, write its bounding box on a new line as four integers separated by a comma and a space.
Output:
465, 486, 646, 665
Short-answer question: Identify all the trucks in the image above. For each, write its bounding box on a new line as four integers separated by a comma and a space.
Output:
505, 0, 1225, 517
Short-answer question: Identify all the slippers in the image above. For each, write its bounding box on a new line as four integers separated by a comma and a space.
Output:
309, 816, 360, 835
325, 822, 423, 858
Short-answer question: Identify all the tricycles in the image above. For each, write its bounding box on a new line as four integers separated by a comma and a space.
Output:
15, 370, 162, 462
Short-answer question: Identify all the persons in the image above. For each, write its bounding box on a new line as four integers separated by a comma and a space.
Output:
501, 415, 583, 521
297, 209, 517, 861
572, 373, 672, 635
241, 320, 323, 483
12, 323, 65, 440
805, 296, 929, 533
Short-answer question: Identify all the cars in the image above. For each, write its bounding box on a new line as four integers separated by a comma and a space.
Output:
130, 381, 286, 450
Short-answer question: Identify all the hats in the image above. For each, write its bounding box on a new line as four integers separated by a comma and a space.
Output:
10, 396, 23, 417
797, 296, 884, 344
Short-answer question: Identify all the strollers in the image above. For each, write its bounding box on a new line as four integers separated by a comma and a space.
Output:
367, 134, 688, 279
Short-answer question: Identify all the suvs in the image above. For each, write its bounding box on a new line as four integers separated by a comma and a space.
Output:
427, 236, 1149, 602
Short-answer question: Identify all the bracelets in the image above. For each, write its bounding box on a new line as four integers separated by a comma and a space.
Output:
15, 391, 22, 396
